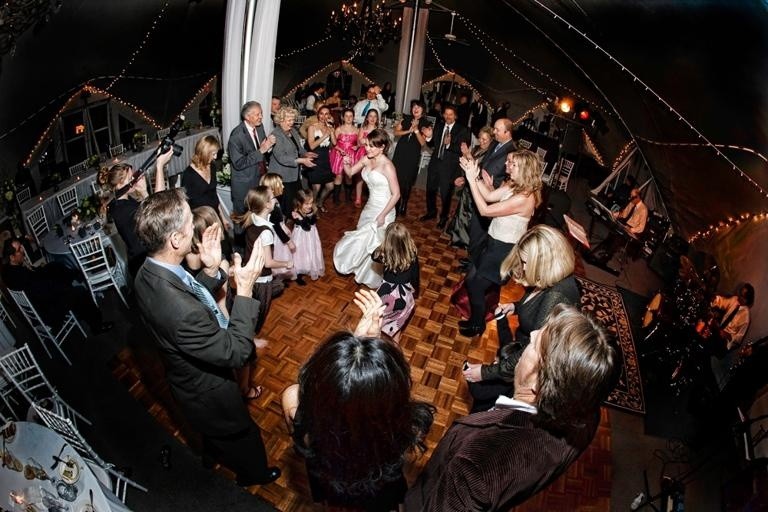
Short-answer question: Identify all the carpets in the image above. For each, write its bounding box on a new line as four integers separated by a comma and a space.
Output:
572, 274, 647, 414
106, 185, 614, 512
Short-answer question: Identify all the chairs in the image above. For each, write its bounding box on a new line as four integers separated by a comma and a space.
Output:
132, 134, 148, 148
68, 161, 87, 177
109, 144, 124, 157
6, 287, 88, 366
91, 180, 111, 205
146, 162, 169, 194
26, 205, 50, 263
418, 149, 434, 174
426, 114, 437, 129
156, 126, 171, 140
540, 162, 549, 176
56, 185, 79, 216
519, 139, 533, 150
541, 162, 563, 189
20, 244, 54, 272
536, 146, 548, 158
30, 402, 148, 504
16, 186, 32, 206
554, 157, 575, 192
0, 342, 92, 431
69, 232, 130, 310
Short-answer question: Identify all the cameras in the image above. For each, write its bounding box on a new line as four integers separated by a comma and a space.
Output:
161, 115, 185, 158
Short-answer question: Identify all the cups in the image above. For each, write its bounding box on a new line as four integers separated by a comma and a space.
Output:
57, 483, 76, 502
3, 451, 16, 469
77, 500, 97, 512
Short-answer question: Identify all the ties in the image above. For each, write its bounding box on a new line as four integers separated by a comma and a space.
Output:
180, 271, 228, 330
253, 129, 268, 177
623, 204, 637, 223
438, 126, 452, 162
357, 102, 371, 130
719, 303, 741, 331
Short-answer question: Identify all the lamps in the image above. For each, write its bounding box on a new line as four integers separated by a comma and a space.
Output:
559, 102, 571, 113
325, 0, 403, 58
574, 100, 590, 120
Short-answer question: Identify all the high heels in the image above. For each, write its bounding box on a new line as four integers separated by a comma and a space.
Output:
458, 321, 474, 328
460, 323, 486, 337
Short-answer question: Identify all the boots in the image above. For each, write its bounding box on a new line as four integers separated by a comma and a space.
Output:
345, 184, 353, 204
333, 184, 342, 206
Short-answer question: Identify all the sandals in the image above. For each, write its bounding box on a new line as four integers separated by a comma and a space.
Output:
237, 385, 262, 400
317, 206, 329, 213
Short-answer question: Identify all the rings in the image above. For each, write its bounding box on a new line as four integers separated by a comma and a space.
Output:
468, 379, 471, 382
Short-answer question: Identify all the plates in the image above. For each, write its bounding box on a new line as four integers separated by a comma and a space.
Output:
59, 455, 80, 485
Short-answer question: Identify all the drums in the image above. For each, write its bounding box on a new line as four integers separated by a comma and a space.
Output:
642, 291, 673, 331
696, 319, 717, 338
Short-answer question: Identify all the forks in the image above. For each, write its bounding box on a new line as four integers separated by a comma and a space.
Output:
52, 455, 74, 468
51, 443, 67, 470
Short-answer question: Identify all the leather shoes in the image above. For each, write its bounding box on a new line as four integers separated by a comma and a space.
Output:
235, 466, 283, 488
419, 212, 438, 221
436, 220, 447, 229
400, 204, 408, 217
459, 256, 470, 264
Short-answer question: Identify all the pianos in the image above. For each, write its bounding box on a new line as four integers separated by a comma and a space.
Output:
579, 196, 636, 242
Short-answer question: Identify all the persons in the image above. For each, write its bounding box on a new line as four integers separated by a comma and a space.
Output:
606, 174, 636, 204
445, 126, 494, 249
302, 106, 336, 213
306, 82, 327, 117
406, 304, 623, 512
372, 222, 421, 344
333, 129, 401, 289
259, 174, 296, 299
279, 289, 437, 512
291, 187, 325, 287
180, 135, 234, 263
0, 237, 115, 336
230, 186, 294, 349
271, 97, 280, 128
420, 103, 468, 229
472, 96, 489, 118
710, 284, 755, 351
354, 108, 380, 208
428, 103, 441, 117
382, 82, 391, 102
538, 115, 551, 137
325, 90, 340, 109
490, 100, 505, 126
458, 151, 542, 338
228, 101, 277, 262
132, 189, 281, 486
299, 103, 334, 137
353, 85, 389, 128
615, 189, 647, 234
107, 164, 173, 289
181, 206, 262, 401
392, 99, 433, 217
450, 149, 528, 323
523, 112, 536, 129
328, 108, 359, 206
461, 227, 583, 415
268, 107, 318, 225
347, 95, 358, 109
452, 119, 518, 274
456, 96, 470, 126
553, 123, 563, 143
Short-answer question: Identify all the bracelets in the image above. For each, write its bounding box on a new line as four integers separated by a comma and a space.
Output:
415, 130, 419, 133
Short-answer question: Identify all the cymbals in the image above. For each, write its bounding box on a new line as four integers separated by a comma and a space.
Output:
680, 254, 699, 282
